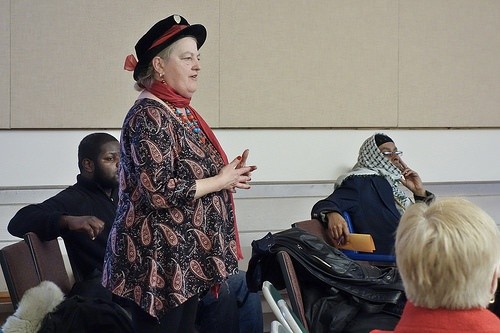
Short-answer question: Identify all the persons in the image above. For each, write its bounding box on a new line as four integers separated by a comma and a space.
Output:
311, 133, 436, 255
370, 198, 500, 333
8, 133, 263, 333
102, 14, 257, 333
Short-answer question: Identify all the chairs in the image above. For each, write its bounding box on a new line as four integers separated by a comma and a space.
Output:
263, 219, 327, 333
0, 233, 74, 310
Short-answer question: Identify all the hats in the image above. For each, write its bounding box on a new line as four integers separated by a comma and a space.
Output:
375, 134, 395, 148
123, 15, 207, 81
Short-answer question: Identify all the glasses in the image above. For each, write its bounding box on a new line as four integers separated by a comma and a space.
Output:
383, 151, 403, 159
